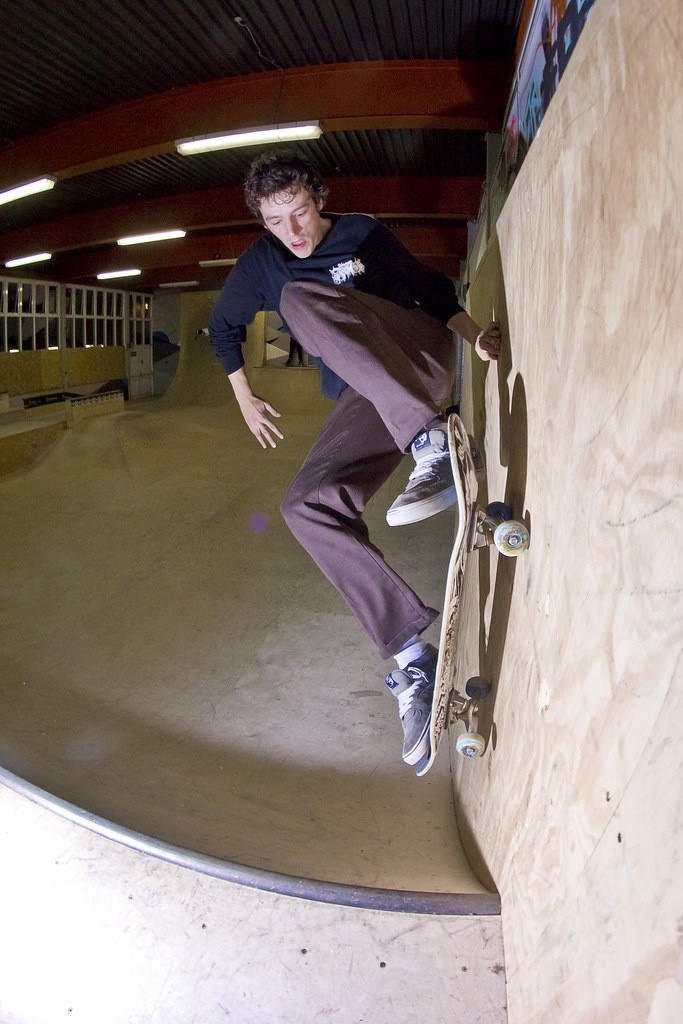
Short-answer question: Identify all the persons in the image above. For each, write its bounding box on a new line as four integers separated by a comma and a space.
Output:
206, 157, 502, 780
487, 1, 590, 224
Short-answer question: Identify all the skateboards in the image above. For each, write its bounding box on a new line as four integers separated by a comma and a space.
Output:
410, 412, 533, 779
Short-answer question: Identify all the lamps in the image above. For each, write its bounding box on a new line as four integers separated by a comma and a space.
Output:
0, 174, 58, 206
199, 259, 237, 267
173, 120, 323, 156
116, 227, 188, 246
4, 251, 53, 267
159, 281, 200, 289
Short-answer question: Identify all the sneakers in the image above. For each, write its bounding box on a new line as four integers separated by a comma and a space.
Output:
386, 428, 486, 527
384, 642, 439, 766
